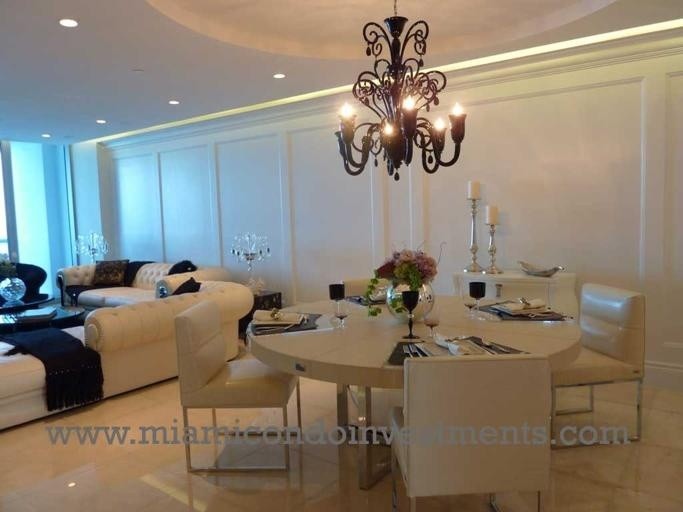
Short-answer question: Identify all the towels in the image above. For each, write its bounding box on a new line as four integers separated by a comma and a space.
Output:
434, 333, 482, 356
503, 297, 553, 318
370, 283, 395, 301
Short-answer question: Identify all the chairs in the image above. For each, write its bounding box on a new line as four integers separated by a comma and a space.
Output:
173, 300, 303, 472
554, 283, 646, 446
388, 355, 551, 508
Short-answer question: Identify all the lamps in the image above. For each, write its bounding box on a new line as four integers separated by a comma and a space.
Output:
495, 264, 497, 265
230, 231, 273, 289
335, 1, 467, 181
72, 232, 109, 267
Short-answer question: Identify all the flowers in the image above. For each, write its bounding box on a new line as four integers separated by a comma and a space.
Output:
364, 248, 438, 316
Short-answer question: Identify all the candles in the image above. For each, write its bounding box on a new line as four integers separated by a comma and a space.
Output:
486, 203, 498, 225
467, 178, 482, 202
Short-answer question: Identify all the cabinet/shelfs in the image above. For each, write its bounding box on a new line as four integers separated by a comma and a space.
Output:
238, 292, 282, 339
453, 267, 577, 323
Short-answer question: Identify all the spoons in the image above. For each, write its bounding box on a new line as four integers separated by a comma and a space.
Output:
481, 336, 511, 354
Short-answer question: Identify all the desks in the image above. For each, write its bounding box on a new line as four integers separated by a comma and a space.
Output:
0, 304, 85, 325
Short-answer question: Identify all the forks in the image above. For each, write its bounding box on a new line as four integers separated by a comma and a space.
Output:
409, 345, 422, 357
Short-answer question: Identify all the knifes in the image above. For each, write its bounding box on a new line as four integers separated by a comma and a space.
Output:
403, 345, 413, 359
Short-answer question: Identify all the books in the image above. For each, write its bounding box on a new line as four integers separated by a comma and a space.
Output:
16, 308, 57, 319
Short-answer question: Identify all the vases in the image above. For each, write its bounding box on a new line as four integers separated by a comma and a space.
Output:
387, 280, 435, 320
0, 278, 26, 307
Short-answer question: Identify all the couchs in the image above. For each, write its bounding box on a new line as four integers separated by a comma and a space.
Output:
0, 279, 255, 430
55, 263, 228, 314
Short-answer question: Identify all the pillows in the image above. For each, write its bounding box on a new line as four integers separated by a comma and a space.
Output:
93, 259, 129, 284
172, 277, 203, 295
168, 260, 197, 274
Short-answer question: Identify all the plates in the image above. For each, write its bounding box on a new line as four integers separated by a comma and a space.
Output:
415, 337, 492, 357
492, 302, 549, 315
518, 260, 563, 276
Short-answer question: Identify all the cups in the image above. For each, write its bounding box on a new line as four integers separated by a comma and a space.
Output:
335, 312, 349, 328
423, 304, 440, 340
463, 295, 477, 312
329, 284, 345, 314
469, 282, 486, 313
402, 291, 420, 339
